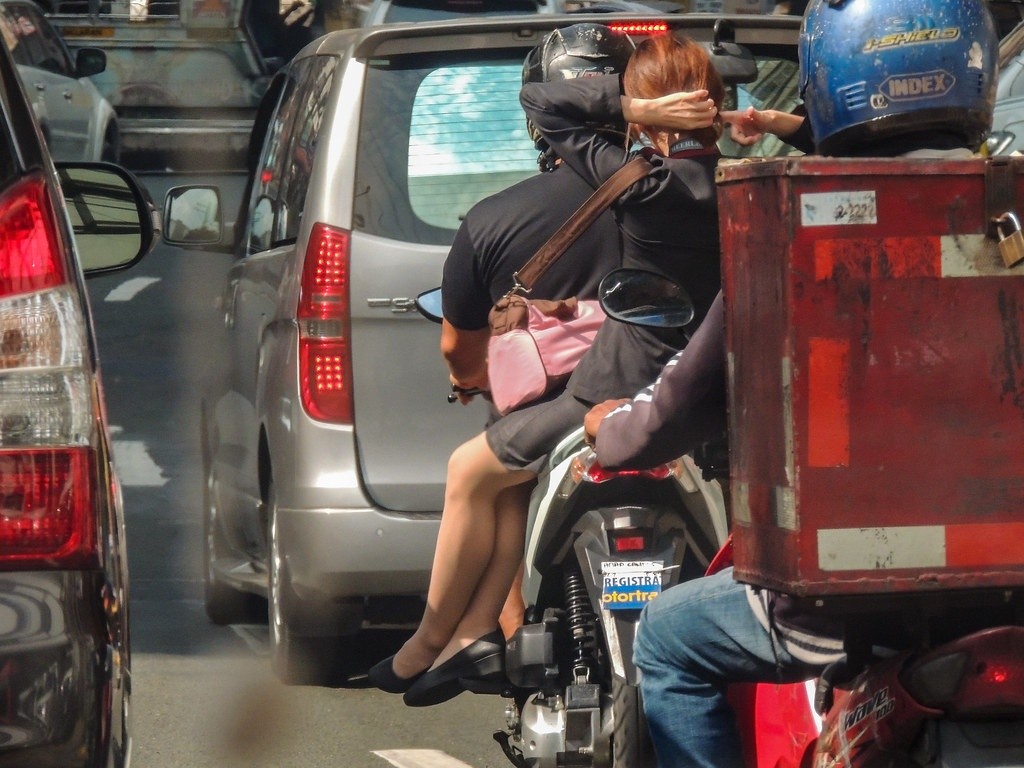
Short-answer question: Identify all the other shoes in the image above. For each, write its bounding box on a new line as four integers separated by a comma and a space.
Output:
368, 654, 434, 694
403, 630, 506, 707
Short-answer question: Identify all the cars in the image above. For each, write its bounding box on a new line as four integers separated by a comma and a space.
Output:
0, 1, 123, 166
45, 0, 1024, 174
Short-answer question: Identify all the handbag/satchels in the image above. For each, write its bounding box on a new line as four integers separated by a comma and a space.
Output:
487, 294, 607, 416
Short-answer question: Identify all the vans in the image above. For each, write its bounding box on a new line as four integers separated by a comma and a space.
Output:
163, 10, 809, 686
1, 18, 161, 767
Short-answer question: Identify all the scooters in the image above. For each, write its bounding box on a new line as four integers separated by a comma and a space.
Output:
413, 281, 700, 768
586, 265, 1024, 768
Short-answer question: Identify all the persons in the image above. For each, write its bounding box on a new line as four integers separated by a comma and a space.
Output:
584, 0, 999, 768
367, 29, 816, 708
440, 19, 636, 642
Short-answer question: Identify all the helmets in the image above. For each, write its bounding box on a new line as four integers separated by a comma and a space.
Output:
522, 23, 636, 149
798, 0, 999, 153
